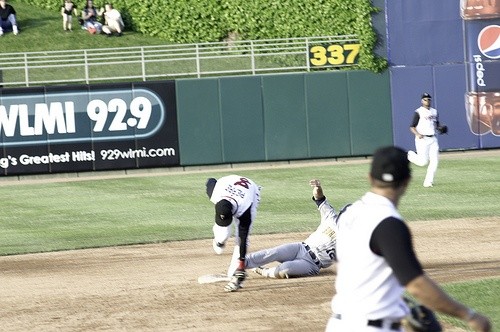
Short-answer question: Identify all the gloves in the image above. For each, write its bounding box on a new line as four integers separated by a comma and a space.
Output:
234, 269, 245, 281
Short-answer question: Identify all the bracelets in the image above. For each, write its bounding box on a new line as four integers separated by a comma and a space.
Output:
464, 310, 478, 322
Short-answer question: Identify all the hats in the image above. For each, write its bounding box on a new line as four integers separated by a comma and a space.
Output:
422, 92, 432, 99
215, 199, 233, 226
339, 204, 352, 214
370, 146, 411, 183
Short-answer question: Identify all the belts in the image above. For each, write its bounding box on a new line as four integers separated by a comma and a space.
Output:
330, 313, 402, 331
302, 242, 322, 269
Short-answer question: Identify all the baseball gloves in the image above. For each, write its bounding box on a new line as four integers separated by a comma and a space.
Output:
437, 126, 449, 135
411, 306, 442, 332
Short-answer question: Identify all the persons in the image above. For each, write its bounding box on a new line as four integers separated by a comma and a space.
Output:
205, 173, 261, 293
59, 0, 77, 34
80, 1, 103, 34
0, 1, 19, 34
327, 147, 492, 332
225, 179, 358, 279
404, 92, 452, 188
99, 2, 123, 36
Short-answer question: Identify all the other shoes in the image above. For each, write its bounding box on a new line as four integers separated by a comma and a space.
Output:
1, 31, 3, 36
107, 32, 113, 37
64, 28, 73, 32
14, 31, 19, 35
116, 32, 122, 37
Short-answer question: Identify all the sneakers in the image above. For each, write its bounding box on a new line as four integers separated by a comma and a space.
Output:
212, 239, 224, 255
251, 265, 270, 278
424, 184, 434, 188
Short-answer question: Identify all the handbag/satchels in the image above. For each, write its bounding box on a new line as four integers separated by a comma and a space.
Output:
84, 18, 105, 35
